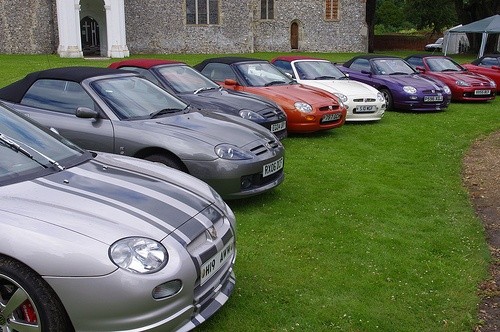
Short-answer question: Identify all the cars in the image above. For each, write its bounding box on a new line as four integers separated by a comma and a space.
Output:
424, 38, 444, 52
247, 55, 386, 123
402, 53, 497, 102
192, 57, 347, 134
460, 55, 500, 92
0, 66, 286, 203
335, 54, 452, 112
0, 100, 239, 332
106, 59, 287, 140
